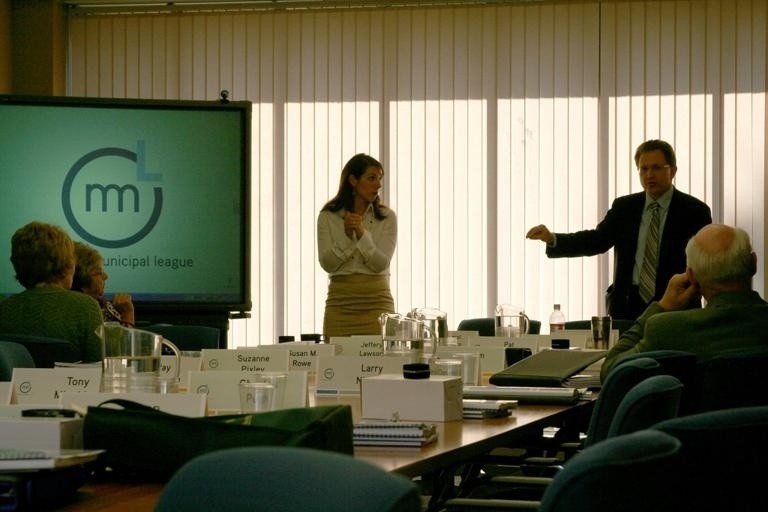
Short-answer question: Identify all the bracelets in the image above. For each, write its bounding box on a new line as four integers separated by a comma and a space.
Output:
118, 319, 134, 328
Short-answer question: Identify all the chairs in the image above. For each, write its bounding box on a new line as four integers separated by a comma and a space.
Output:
433, 429, 682, 512
8, 335, 81, 381
458, 315, 495, 337
520, 358, 661, 453
471, 373, 682, 493
0, 343, 34, 387
547, 349, 697, 443
525, 319, 540, 335
679, 345, 767, 415
565, 320, 590, 332
157, 445, 423, 511
653, 409, 768, 512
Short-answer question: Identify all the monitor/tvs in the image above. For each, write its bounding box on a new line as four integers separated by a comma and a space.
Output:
2, 97, 253, 307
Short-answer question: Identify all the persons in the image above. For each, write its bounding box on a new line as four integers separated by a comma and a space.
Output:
0, 220, 106, 363
70, 241, 135, 328
317, 153, 398, 343
526, 139, 713, 319
600, 223, 768, 388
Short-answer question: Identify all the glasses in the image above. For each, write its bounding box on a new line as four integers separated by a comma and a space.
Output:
637, 162, 674, 174
90, 267, 106, 279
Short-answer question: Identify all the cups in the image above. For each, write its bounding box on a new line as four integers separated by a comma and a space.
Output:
301, 333, 327, 345
255, 372, 288, 410
551, 338, 571, 349
238, 382, 273, 416
505, 347, 532, 367
279, 335, 294, 343
451, 353, 480, 387
434, 359, 461, 377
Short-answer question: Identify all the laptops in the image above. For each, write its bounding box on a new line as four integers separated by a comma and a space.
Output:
489, 348, 605, 391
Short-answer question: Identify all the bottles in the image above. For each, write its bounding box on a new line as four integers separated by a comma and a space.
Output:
591, 318, 611, 351
550, 303, 564, 331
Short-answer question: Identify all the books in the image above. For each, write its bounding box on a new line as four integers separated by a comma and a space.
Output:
352, 418, 439, 449
461, 397, 521, 420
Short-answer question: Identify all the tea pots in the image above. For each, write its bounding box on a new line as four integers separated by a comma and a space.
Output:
494, 303, 530, 338
406, 307, 448, 344
95, 320, 181, 394
378, 312, 437, 356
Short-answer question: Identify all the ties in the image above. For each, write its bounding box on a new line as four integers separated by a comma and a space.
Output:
637, 201, 661, 305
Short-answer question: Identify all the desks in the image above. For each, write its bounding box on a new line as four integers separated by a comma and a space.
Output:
48, 375, 598, 512
